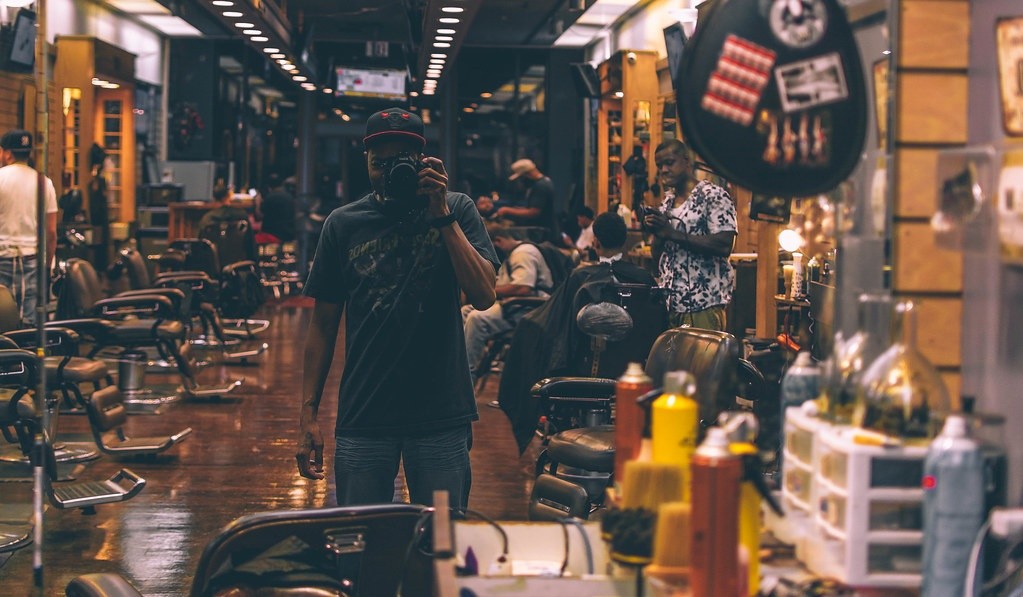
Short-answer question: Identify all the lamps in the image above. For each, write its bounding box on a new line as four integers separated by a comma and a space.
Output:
549, 18, 564, 35
409, 79, 420, 97
321, 81, 334, 94
568, 0, 585, 12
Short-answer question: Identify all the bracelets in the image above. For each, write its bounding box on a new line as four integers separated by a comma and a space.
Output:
431, 210, 457, 228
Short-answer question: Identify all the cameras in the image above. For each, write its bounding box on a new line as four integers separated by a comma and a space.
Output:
382, 151, 430, 200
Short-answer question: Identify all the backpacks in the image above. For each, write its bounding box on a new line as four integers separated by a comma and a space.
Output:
505, 241, 576, 296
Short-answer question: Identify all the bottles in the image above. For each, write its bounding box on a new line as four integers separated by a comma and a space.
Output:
807, 257, 820, 294
851, 299, 952, 443
820, 288, 892, 424
922, 415, 987, 597
688, 411, 759, 597
612, 359, 698, 500
781, 350, 820, 495
781, 253, 803, 299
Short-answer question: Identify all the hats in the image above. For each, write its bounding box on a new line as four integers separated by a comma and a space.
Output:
0, 130, 35, 152
508, 159, 536, 180
363, 107, 425, 152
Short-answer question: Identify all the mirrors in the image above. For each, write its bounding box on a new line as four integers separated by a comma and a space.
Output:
0, 0, 974, 578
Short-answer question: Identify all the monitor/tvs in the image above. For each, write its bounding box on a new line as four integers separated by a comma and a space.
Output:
663, 21, 688, 89
3, 7, 38, 74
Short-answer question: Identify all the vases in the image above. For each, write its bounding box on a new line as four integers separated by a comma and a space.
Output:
790, 252, 802, 300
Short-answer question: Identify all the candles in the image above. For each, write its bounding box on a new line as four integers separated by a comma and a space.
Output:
783, 264, 794, 282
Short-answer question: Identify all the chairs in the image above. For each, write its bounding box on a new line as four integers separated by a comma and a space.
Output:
0, 207, 829, 597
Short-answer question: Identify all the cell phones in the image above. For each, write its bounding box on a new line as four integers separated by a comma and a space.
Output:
561, 233, 567, 237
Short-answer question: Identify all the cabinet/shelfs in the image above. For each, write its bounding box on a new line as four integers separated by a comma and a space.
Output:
780, 405, 853, 561
596, 50, 659, 216
53, 35, 139, 224
813, 403, 1006, 589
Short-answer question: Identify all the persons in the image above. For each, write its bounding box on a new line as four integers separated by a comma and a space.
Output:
215, 176, 330, 294
295, 105, 501, 517
461, 139, 739, 457
0, 129, 58, 327
